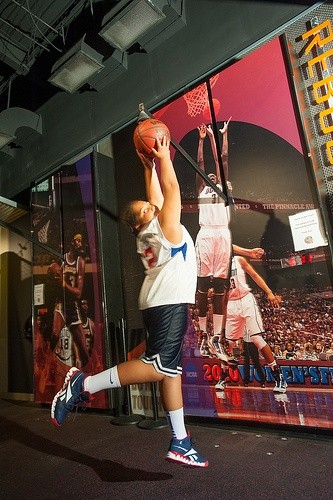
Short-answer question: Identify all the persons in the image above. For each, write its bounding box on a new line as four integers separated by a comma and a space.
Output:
183, 116, 333, 399
34, 233, 96, 407
51, 135, 211, 468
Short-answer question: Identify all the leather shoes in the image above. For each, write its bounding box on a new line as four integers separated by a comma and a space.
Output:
244, 383, 249, 387
260, 383, 265, 388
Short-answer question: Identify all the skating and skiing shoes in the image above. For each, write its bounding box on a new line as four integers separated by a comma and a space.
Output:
163, 435, 209, 468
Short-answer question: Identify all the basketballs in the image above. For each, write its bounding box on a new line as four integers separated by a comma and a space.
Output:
133, 119, 171, 160
47, 263, 61, 280
202, 98, 220, 121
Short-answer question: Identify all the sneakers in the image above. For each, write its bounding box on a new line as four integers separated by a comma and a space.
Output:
49, 366, 92, 432
272, 373, 288, 393
199, 331, 229, 362
214, 378, 228, 391
